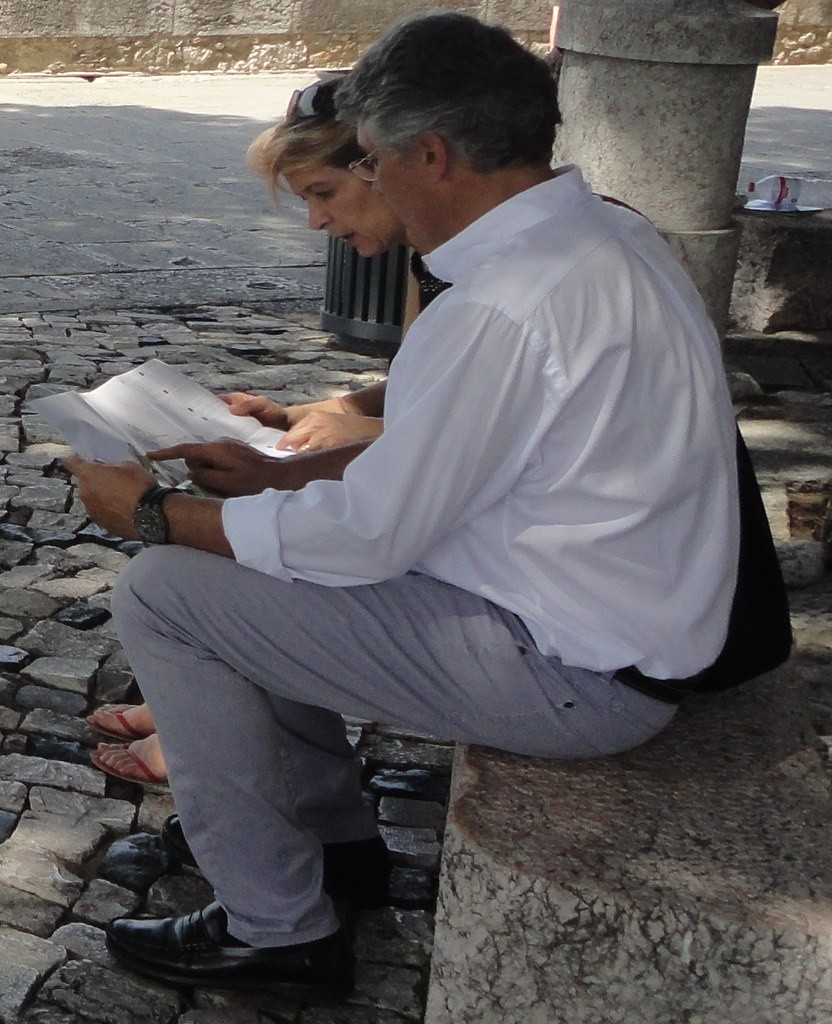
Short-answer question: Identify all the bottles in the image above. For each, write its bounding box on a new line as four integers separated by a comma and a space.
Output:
749, 176, 832, 208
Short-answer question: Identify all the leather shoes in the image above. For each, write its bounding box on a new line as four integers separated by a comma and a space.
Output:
162, 813, 392, 902
106, 900, 355, 1003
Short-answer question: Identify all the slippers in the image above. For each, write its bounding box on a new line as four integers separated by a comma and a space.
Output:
86, 705, 148, 742
89, 743, 171, 793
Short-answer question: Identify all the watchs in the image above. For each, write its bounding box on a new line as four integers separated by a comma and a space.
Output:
133, 484, 183, 549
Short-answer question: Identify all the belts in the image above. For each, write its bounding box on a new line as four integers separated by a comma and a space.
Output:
616, 666, 684, 705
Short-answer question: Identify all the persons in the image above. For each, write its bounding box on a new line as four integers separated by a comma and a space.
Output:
60, 11, 741, 985
85, 77, 452, 786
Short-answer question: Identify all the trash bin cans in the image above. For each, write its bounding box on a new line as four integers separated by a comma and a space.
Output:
311, 69, 425, 354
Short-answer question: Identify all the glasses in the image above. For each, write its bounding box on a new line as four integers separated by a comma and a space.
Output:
287, 85, 338, 122
349, 147, 380, 181
